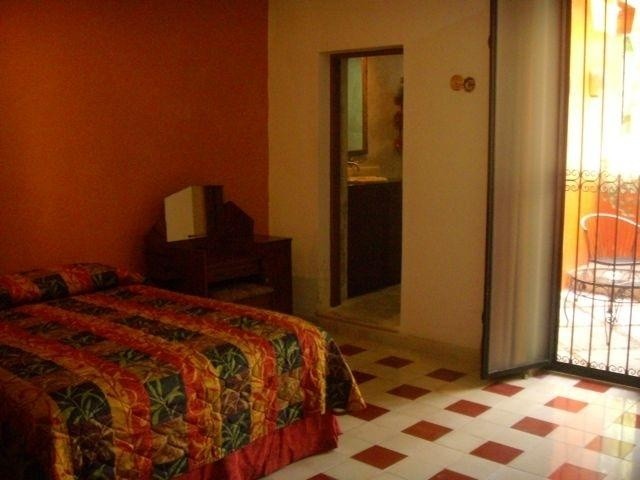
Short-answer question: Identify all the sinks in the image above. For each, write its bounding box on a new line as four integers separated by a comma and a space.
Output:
348, 175, 385, 182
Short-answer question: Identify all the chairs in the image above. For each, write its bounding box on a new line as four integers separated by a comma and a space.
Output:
581, 211, 640, 267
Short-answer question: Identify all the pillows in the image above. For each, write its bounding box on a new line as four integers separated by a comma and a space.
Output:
0, 261, 145, 308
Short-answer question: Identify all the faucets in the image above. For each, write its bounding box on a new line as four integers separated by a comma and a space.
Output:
347, 161, 361, 172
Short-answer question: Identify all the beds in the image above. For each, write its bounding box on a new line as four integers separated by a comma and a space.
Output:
0, 281, 363, 478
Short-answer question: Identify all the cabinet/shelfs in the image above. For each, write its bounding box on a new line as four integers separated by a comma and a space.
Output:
348, 182, 400, 298
151, 234, 295, 314
